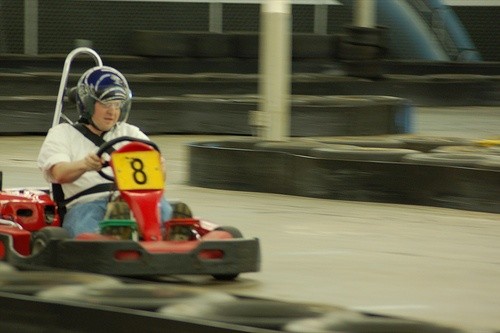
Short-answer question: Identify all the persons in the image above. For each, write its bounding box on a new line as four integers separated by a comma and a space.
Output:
37, 66, 174, 243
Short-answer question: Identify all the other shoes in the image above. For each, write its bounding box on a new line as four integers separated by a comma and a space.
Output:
101, 202, 133, 240
164, 202, 192, 241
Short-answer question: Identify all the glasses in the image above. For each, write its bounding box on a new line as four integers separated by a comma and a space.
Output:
93, 96, 122, 109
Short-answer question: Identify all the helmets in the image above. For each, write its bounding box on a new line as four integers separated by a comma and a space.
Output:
76, 66, 130, 119
